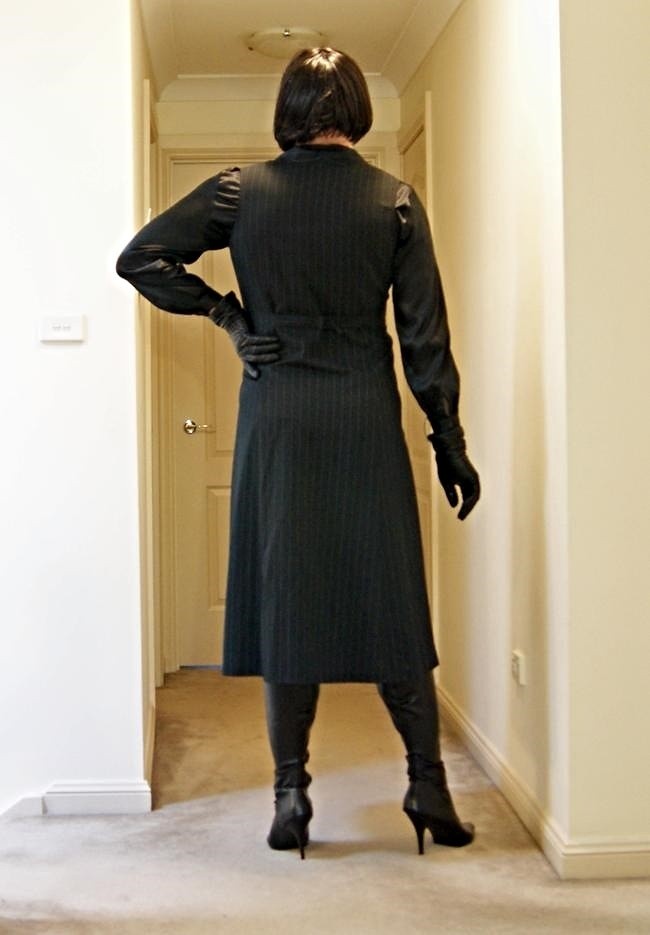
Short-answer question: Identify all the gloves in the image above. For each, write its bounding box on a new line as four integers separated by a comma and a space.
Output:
209, 290, 280, 380
428, 427, 481, 520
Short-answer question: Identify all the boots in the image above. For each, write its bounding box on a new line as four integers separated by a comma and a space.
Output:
265, 677, 319, 859
377, 671, 474, 855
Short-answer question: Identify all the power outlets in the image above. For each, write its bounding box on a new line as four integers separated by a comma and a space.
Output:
510, 651, 525, 686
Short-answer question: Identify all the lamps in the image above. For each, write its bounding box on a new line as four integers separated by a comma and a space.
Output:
246, 24, 329, 59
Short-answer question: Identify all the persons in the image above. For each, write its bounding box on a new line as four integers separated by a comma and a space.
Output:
115, 47, 481, 863
